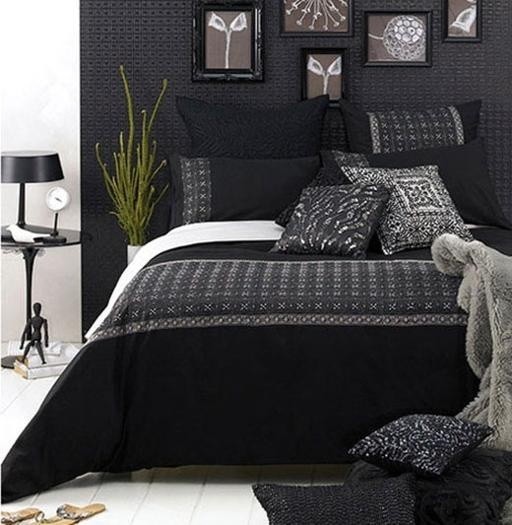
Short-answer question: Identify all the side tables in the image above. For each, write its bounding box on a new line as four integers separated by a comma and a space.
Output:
1, 227, 94, 357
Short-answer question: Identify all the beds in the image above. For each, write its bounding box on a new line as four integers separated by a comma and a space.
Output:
0, 214, 512, 525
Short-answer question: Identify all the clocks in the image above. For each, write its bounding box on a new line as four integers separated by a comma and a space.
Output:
43, 187, 72, 244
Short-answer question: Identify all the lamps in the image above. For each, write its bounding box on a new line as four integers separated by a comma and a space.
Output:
1, 152, 64, 241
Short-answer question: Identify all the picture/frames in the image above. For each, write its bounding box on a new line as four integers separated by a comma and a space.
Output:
440, 0, 485, 46
190, 0, 267, 85
361, 8, 433, 70
278, 0, 356, 39
300, 46, 349, 106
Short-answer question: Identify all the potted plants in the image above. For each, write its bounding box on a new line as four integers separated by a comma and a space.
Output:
95, 64, 170, 267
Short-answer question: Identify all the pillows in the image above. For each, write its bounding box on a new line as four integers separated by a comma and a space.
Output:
250, 481, 418, 525
173, 93, 331, 155
350, 448, 512, 525
341, 163, 475, 257
320, 135, 512, 230
338, 96, 490, 154
173, 155, 321, 235
268, 183, 397, 264
348, 411, 497, 481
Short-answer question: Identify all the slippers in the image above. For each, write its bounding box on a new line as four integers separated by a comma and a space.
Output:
1, 507, 40, 523
44, 502, 106, 523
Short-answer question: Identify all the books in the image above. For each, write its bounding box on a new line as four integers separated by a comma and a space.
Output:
13, 353, 73, 380
